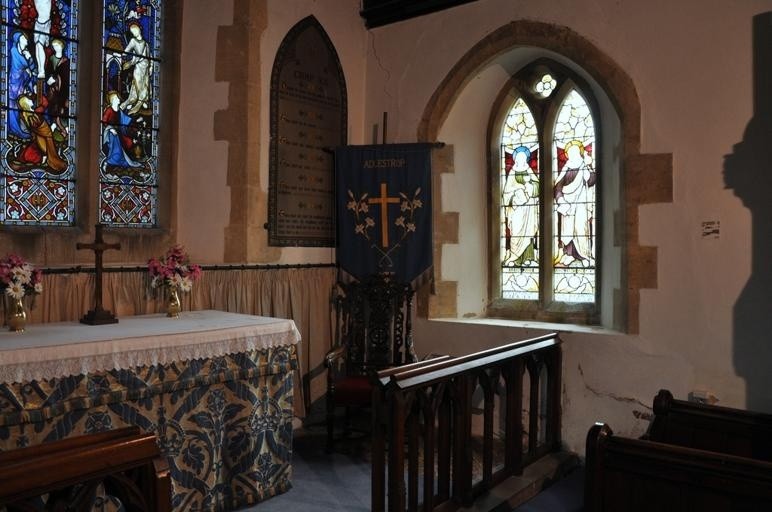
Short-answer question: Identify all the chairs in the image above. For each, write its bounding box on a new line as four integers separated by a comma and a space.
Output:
321, 272, 424, 462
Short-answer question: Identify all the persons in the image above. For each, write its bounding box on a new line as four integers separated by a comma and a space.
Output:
502, 146, 540, 267
102, 19, 152, 168
6, 0, 71, 176
554, 140, 596, 268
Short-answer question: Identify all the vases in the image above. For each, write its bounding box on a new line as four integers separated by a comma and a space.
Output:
164, 283, 181, 319
8, 297, 27, 335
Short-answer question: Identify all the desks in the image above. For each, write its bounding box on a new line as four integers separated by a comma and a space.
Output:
0, 309, 298, 512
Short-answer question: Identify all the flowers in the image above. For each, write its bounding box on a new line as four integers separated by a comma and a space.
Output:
145, 244, 202, 291
1, 251, 44, 299
